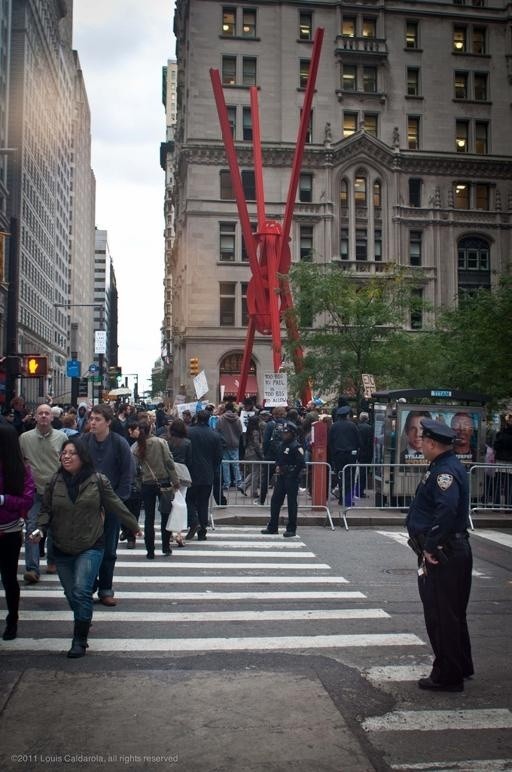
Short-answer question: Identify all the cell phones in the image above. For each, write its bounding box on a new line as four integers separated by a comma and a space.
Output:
31, 529, 40, 538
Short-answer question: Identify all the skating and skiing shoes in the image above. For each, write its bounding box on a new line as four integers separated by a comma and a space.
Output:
283, 531, 295, 537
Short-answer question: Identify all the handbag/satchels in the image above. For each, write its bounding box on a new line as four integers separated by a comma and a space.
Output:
158, 487, 174, 513
172, 461, 193, 488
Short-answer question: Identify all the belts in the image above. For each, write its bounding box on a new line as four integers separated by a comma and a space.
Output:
280, 465, 297, 468
450, 532, 466, 540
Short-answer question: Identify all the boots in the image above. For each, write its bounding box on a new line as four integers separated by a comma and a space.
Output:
68, 620, 90, 657
3, 588, 19, 640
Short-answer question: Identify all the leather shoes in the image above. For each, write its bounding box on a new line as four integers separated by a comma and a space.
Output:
120, 523, 207, 559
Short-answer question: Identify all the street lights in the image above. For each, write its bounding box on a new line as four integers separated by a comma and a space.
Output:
48, 301, 113, 406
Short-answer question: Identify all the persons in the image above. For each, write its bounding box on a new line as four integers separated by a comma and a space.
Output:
400, 411, 433, 473
370, 414, 397, 481
483, 413, 512, 515
404, 416, 477, 693
1, 394, 375, 657
449, 413, 478, 475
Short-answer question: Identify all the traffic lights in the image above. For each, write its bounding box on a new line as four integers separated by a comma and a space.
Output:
189, 357, 199, 375
22, 355, 47, 379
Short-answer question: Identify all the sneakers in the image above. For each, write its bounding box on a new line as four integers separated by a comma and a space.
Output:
262, 529, 278, 534
418, 678, 464, 692
46, 564, 57, 574
332, 486, 366, 506
24, 571, 39, 582
464, 668, 473, 677
100, 597, 116, 605
222, 481, 264, 505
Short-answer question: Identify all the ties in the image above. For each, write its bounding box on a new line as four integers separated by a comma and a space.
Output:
414, 451, 422, 458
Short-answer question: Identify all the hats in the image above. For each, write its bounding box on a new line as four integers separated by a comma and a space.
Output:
418, 419, 457, 445
337, 406, 351, 414
276, 422, 298, 433
51, 407, 63, 417
